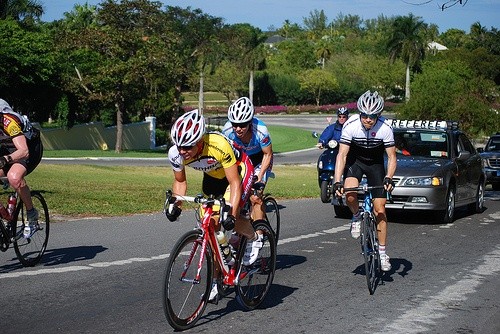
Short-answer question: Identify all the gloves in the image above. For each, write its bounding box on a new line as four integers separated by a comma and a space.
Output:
0, 155, 12, 169
221, 215, 236, 231
165, 207, 182, 222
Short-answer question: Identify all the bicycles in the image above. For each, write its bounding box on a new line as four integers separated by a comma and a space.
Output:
327, 183, 394, 295
159, 189, 277, 331
251, 182, 281, 245
0, 161, 49, 267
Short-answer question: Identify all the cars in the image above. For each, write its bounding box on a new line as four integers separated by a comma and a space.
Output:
331, 118, 488, 224
477, 132, 500, 185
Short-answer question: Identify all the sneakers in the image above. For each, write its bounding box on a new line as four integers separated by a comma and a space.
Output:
379, 251, 392, 271
350, 214, 362, 239
243, 232, 263, 265
200, 278, 218, 300
23, 209, 40, 239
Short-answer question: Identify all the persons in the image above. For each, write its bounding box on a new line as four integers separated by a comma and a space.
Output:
0, 97, 43, 239
165, 96, 273, 303
332, 91, 397, 271
315, 107, 348, 149
395, 135, 411, 155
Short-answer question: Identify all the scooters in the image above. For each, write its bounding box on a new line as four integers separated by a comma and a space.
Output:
311, 131, 340, 204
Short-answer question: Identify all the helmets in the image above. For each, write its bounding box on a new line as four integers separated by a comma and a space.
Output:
171, 109, 206, 147
357, 90, 384, 117
228, 97, 254, 123
337, 107, 348, 116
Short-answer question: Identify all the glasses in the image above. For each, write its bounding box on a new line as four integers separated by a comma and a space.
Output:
339, 115, 346, 118
175, 146, 193, 151
231, 121, 249, 128
361, 113, 378, 119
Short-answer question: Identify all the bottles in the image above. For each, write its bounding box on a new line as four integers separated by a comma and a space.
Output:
216, 231, 229, 255
0, 202, 8, 220
6, 194, 17, 220
226, 233, 240, 265
